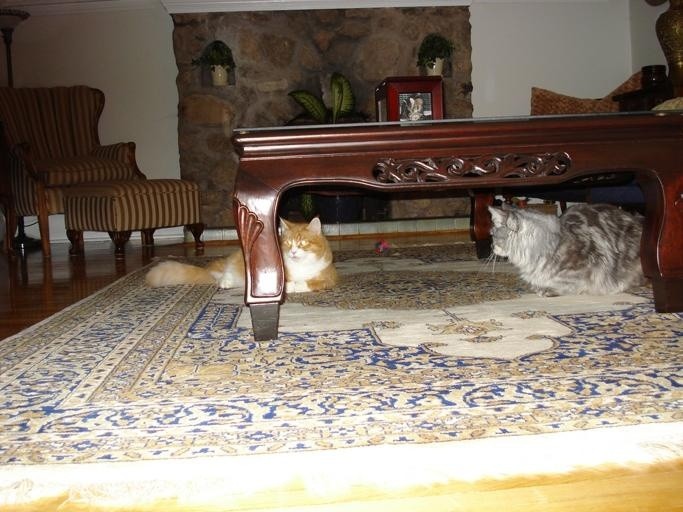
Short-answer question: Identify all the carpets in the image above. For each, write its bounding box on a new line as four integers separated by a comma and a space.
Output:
1, 241, 679, 512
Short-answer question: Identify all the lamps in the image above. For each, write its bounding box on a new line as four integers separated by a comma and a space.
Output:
0, 9, 42, 250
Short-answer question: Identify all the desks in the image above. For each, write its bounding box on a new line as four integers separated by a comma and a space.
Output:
219, 107, 681, 339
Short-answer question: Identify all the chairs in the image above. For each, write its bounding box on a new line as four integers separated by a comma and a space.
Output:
2, 84, 145, 258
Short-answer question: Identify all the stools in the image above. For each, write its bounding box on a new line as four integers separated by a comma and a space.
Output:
58, 177, 206, 259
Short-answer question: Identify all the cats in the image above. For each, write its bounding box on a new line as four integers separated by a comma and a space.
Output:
475, 201, 651, 296
144, 217, 337, 294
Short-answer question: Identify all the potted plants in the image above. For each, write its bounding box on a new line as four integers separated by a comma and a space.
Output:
196, 40, 234, 87
416, 34, 452, 77
283, 73, 375, 222
